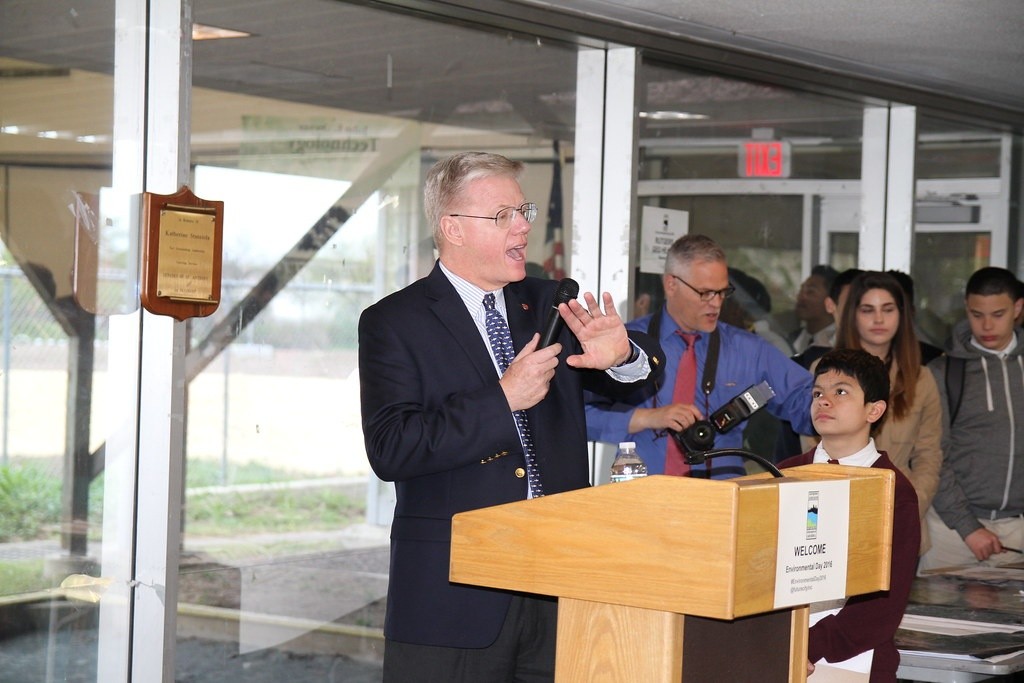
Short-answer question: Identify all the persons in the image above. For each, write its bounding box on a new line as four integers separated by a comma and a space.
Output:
785, 265, 840, 359
775, 348, 921, 683
773, 269, 863, 461
358, 152, 667, 683
917, 266, 1024, 577
583, 234, 815, 480
808, 268, 944, 557
719, 267, 793, 359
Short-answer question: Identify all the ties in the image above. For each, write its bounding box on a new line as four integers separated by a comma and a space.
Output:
664, 330, 701, 478
483, 293, 545, 499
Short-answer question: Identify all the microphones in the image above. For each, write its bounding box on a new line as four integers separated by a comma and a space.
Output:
533, 277, 578, 353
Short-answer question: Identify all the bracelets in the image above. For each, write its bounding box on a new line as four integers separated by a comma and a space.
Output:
618, 343, 637, 368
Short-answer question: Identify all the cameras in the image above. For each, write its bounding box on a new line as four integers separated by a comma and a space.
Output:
666, 379, 776, 465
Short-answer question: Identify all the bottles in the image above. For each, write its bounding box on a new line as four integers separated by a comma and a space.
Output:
610, 441, 648, 484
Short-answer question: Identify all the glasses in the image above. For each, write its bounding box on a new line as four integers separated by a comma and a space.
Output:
672, 275, 736, 301
449, 201, 538, 229
